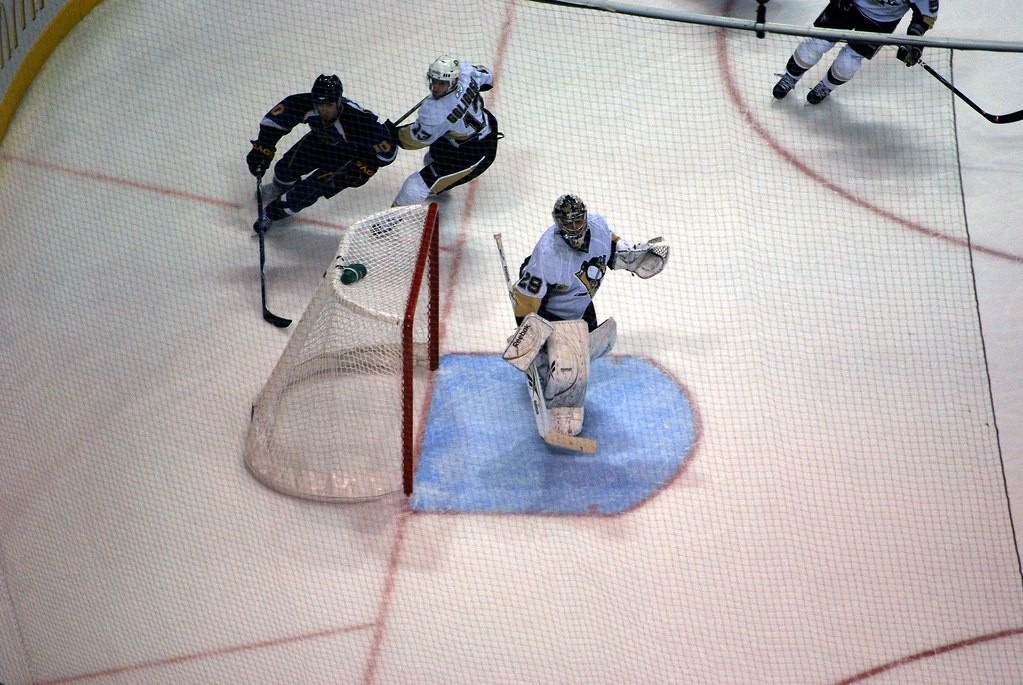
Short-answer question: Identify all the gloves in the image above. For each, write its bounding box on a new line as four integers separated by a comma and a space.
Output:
246, 139, 277, 178
380, 119, 397, 141
896, 20, 930, 67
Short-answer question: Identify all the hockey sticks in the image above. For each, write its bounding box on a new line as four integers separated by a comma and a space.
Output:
493, 231, 598, 454
256, 163, 293, 328
918, 58, 1023, 124
393, 94, 431, 127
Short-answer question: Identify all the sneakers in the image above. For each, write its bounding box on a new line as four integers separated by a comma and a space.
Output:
251, 207, 273, 238
770, 71, 804, 103
370, 203, 405, 239
254, 170, 297, 206
804, 75, 840, 107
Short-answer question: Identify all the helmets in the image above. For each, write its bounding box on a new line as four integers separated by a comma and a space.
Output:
552, 193, 589, 249
311, 74, 343, 121
427, 54, 462, 99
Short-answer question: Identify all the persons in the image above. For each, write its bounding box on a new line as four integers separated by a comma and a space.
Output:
246, 72, 398, 238
372, 55, 499, 240
771, 0, 940, 106
503, 194, 669, 436
